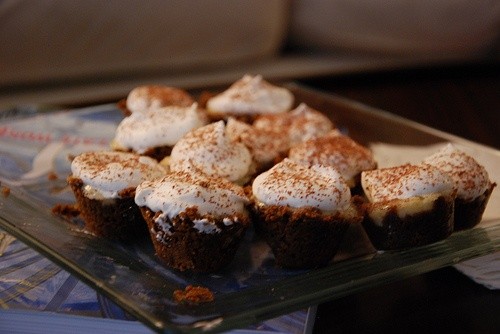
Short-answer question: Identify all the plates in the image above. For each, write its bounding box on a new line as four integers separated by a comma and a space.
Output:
0, 181, 500, 333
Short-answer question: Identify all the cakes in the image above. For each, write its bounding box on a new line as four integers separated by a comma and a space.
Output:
67, 74, 496, 273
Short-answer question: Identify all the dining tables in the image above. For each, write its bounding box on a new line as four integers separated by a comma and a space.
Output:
0, 55, 499, 333
